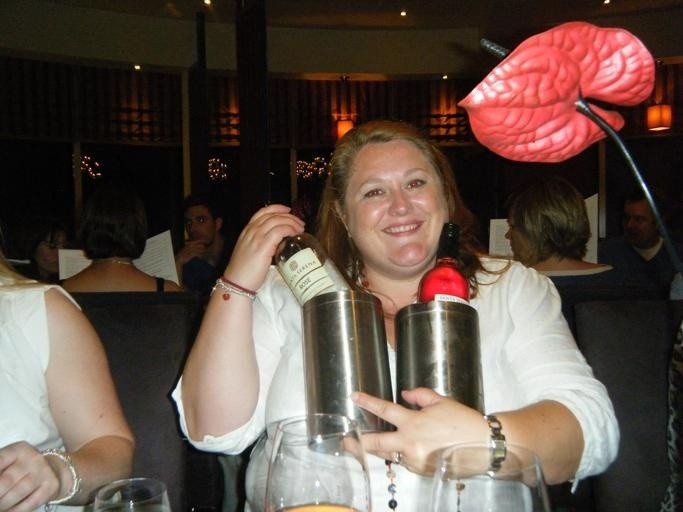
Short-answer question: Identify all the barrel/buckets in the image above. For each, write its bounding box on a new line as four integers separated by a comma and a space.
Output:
393, 299, 483, 417
300, 293, 393, 455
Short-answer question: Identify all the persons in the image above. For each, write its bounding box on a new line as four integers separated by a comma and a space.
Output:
20, 195, 236, 290
505, 172, 621, 289
173, 117, 621, 512
600, 186, 683, 296
0, 252, 136, 512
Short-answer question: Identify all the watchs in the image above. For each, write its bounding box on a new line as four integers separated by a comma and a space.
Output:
484, 415, 506, 476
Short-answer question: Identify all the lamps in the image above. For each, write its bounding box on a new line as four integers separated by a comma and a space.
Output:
646, 103, 672, 133
336, 118, 354, 139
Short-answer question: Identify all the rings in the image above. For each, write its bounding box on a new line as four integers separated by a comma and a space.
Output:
394, 452, 401, 464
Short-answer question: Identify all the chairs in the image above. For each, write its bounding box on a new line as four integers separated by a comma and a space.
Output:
560, 283, 683, 512
72, 295, 211, 511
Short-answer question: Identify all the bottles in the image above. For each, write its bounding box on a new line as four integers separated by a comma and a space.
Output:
256, 194, 352, 307
415, 219, 471, 307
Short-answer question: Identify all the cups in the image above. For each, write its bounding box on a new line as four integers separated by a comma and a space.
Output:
429, 440, 552, 512
93, 479, 173, 512
260, 412, 375, 512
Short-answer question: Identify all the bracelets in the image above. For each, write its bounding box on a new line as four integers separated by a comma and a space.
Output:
41, 448, 81, 504
216, 276, 258, 301
